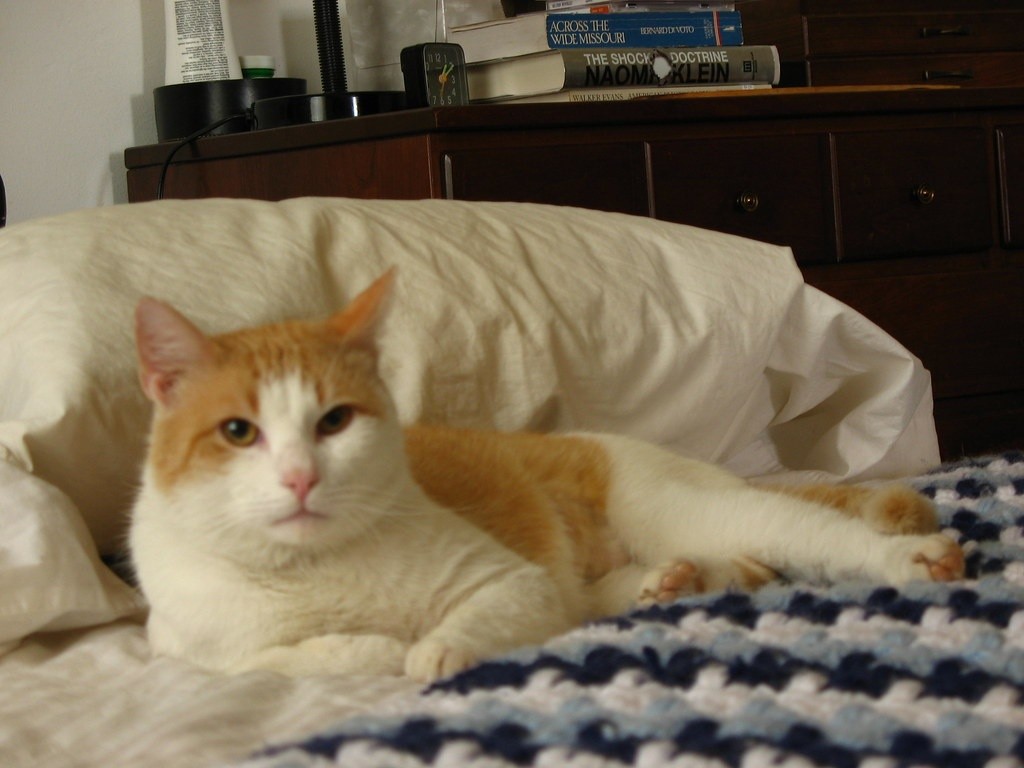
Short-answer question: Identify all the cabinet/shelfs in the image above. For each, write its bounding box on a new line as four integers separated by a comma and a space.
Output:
125, 86, 1024, 459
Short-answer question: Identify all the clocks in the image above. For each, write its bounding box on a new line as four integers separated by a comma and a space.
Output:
400, 42, 470, 110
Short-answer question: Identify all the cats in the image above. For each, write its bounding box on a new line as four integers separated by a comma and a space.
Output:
128, 262, 965, 685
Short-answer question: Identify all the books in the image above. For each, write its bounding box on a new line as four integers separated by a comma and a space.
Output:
445, 0, 780, 101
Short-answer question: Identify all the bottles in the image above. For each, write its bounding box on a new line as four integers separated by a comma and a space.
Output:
164, 0, 243, 86
